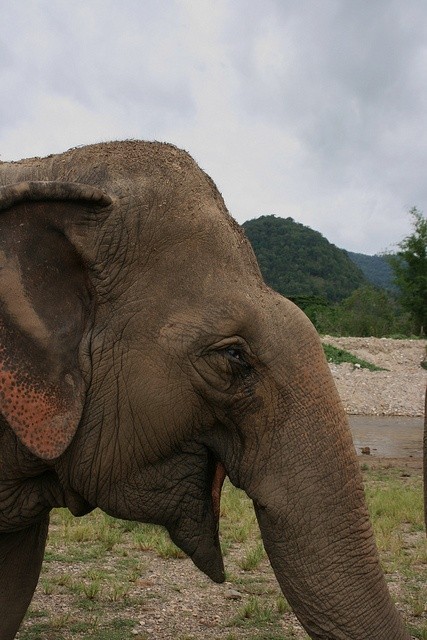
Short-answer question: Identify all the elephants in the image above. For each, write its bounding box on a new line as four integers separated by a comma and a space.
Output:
0, 136, 416, 640
